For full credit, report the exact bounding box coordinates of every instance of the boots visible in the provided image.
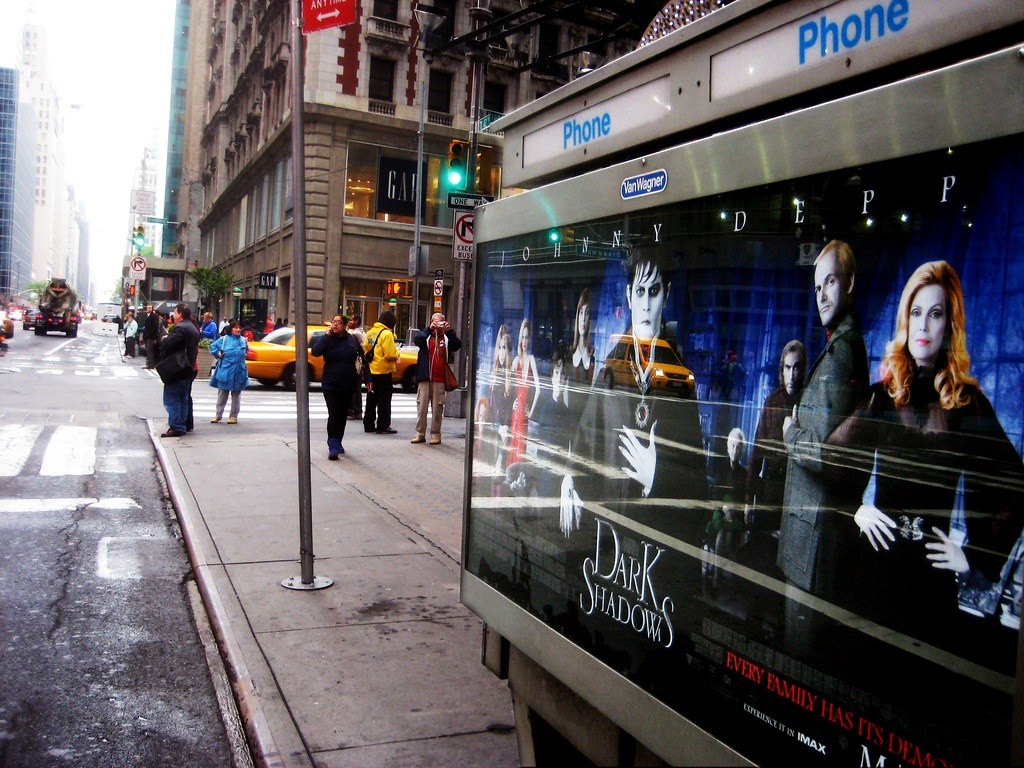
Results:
[327,438,338,459]
[337,441,344,454]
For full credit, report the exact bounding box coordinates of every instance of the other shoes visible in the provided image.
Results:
[227,418,237,423]
[365,427,377,432]
[346,414,362,420]
[430,438,441,444]
[410,437,426,443]
[211,416,221,423]
[376,424,397,434]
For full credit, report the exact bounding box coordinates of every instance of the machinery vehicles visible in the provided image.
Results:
[34,277,82,338]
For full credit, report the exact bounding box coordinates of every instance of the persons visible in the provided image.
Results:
[708,428,749,598]
[475,288,595,520]
[822,261,1024,613]
[219,317,234,335]
[199,312,217,341]
[263,317,288,333]
[363,311,400,434]
[311,314,372,460]
[209,320,249,423]
[411,313,462,445]
[142,305,159,369]
[347,313,366,420]
[775,241,871,656]
[561,235,707,613]
[123,309,138,358]
[160,303,202,438]
[746,340,807,578]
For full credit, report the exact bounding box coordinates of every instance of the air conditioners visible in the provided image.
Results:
[413,2,448,43]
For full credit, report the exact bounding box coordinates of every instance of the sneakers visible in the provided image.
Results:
[161,428,186,437]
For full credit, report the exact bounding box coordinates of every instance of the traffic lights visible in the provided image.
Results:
[131,226,138,245]
[549,231,560,244]
[391,280,407,295]
[446,142,464,187]
[561,227,575,245]
[137,224,145,247]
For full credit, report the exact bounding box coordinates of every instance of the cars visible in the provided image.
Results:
[0,310,15,343]
[22,308,38,330]
[245,323,421,393]
[102,314,121,324]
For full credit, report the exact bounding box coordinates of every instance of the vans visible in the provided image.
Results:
[599,333,696,399]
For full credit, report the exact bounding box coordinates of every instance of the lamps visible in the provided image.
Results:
[505,22,532,50]
[581,48,602,71]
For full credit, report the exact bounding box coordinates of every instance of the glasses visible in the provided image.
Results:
[330,320,344,324]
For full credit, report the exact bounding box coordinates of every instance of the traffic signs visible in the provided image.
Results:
[447,192,495,212]
[301,0,357,36]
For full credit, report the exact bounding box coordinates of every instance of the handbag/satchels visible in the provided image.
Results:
[444,363,457,391]
[156,351,192,384]
[209,365,219,388]
[350,333,362,375]
[365,349,374,362]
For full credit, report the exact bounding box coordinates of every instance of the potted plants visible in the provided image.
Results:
[194,339,214,379]
